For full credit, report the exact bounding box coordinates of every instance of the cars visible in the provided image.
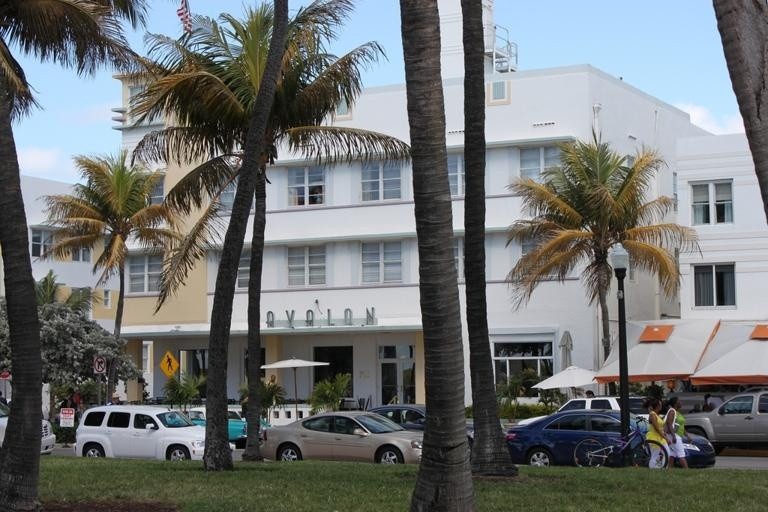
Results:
[167,405,268,443]
[1,395,56,455]
[259,411,424,464]
[370,405,473,445]
[507,398,717,468]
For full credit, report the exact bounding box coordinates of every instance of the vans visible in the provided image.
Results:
[74,404,236,462]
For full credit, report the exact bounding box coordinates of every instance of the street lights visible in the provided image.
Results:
[610,242,635,465]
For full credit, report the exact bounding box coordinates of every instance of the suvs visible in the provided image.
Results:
[684,387,767,457]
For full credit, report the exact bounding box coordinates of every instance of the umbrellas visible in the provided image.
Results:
[259,354,331,422]
[529,365,598,398]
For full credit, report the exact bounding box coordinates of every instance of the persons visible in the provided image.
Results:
[106,392,122,406]
[640,397,672,470]
[687,403,700,413]
[265,374,276,405]
[703,393,723,410]
[0,391,6,405]
[585,390,596,398]
[661,396,688,469]
